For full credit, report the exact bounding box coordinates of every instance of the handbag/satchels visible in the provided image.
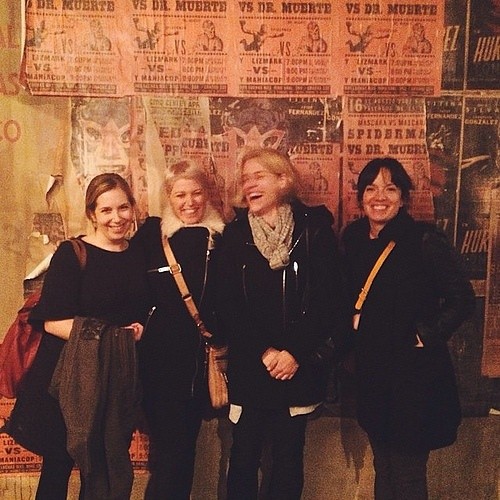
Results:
[285,335,340,419]
[334,330,370,410]
[0,236,89,400]
[208,346,229,409]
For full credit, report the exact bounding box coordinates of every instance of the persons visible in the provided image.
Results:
[51,162,227,500]
[328,157,476,500]
[217,148,338,500]
[4,173,146,500]
